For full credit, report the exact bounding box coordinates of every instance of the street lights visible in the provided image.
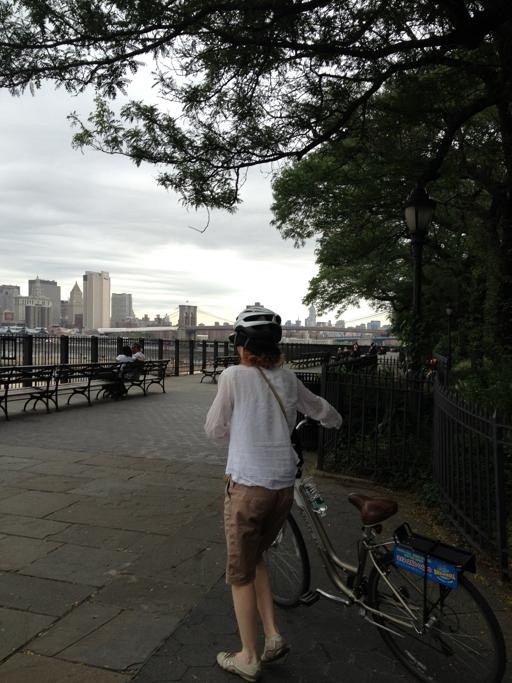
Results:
[444,301,455,390]
[402,178,436,433]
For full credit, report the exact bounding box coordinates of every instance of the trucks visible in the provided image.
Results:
[196,334,209,342]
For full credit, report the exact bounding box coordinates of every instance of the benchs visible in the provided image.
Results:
[0,358,171,421]
[289,347,388,369]
[201,354,239,383]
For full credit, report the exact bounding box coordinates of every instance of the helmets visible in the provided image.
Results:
[229,304,282,342]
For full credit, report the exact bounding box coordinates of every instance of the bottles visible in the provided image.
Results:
[299,475,329,519]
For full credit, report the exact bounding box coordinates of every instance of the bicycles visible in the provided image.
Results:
[260,415,506,682]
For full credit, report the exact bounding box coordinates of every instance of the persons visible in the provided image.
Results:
[336,340,388,353]
[202,302,346,681]
[101,344,133,399]
[131,342,145,363]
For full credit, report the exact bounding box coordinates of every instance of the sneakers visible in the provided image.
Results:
[216,651,262,682]
[260,634,290,664]
